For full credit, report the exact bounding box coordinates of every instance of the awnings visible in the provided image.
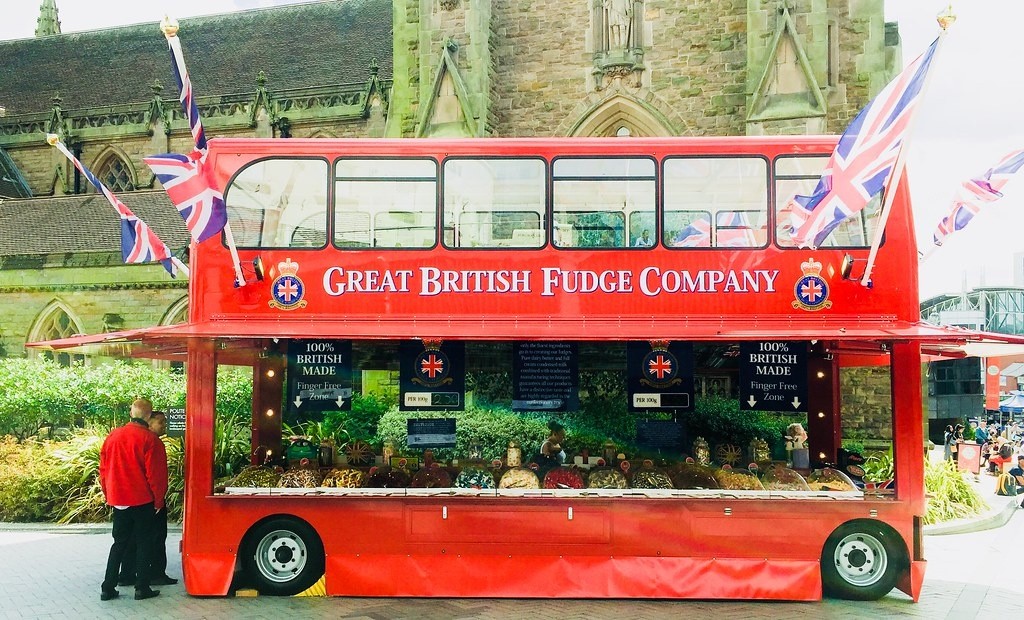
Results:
[144,319,1024,346]
[28,322,188,362]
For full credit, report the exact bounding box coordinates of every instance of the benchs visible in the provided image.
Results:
[1002,462,1017,476]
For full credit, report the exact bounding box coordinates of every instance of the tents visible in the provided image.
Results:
[985,395,1024,425]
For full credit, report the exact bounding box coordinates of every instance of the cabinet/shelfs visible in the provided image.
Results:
[956,443,982,474]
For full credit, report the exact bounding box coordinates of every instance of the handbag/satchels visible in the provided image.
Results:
[950,445,957,453]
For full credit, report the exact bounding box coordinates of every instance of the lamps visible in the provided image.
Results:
[841,253,867,282]
[240,255,265,281]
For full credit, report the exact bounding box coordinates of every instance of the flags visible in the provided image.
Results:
[778,188,804,246]
[142,74,228,244]
[674,211,753,247]
[789,36,939,250]
[935,149,1024,238]
[71,156,177,280]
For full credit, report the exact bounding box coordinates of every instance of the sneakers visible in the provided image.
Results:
[148,574,178,585]
[118,577,136,586]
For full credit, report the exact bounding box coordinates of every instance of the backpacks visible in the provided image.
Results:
[996,473,1017,495]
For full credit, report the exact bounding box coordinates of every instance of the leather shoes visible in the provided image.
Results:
[100,589,120,600]
[134,589,160,600]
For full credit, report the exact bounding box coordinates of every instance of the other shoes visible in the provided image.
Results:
[981,464,986,467]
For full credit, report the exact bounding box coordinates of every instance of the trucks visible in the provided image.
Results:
[24,134,927,603]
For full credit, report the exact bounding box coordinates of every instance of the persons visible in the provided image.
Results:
[980,420,1024,475]
[98,399,169,601]
[1008,455,1024,509]
[952,424,965,460]
[944,425,953,460]
[539,420,567,462]
[635,230,652,247]
[120,411,178,585]
[975,419,989,443]
